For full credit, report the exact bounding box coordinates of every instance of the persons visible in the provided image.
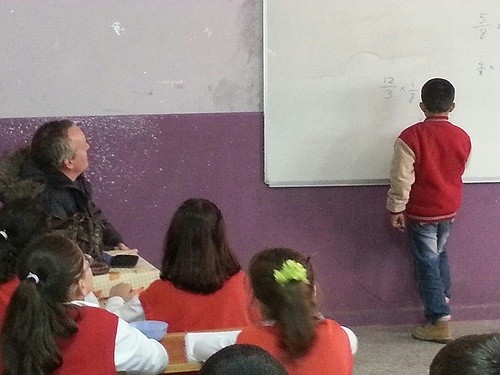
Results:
[0,233,168,375]
[192,247,358,375]
[106,198,267,335]
[198,344,290,375]
[386,77,472,343]
[1,119,139,277]
[0,196,101,327]
[429,332,500,375]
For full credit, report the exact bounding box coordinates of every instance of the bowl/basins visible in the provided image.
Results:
[129,320,169,342]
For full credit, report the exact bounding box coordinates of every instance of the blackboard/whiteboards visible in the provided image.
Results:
[263,0,500,184]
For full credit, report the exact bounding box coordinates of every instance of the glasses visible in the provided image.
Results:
[76,252,94,284]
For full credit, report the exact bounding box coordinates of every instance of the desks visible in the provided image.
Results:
[147,330,240,375]
[87,251,161,309]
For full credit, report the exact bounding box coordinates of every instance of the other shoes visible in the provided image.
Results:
[413,320,453,344]
